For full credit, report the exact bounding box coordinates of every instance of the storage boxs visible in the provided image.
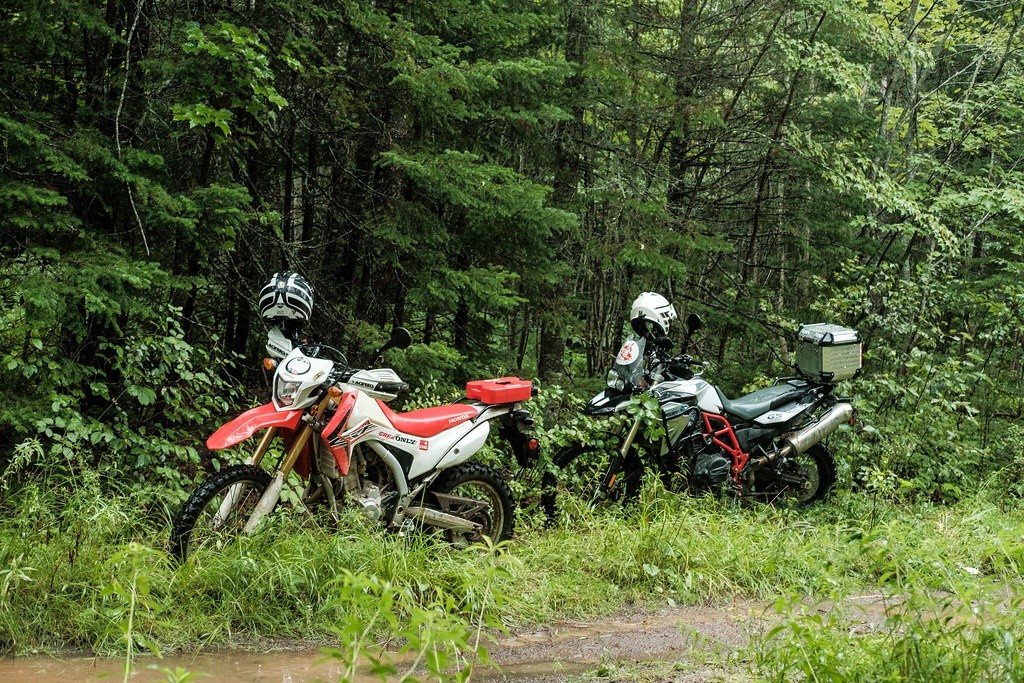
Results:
[795,322,862,381]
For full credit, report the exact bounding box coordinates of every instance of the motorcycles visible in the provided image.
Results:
[168,319,543,566]
[540,320,864,524]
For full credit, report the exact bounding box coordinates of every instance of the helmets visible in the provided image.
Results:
[629,291,678,343]
[258,270,315,330]
